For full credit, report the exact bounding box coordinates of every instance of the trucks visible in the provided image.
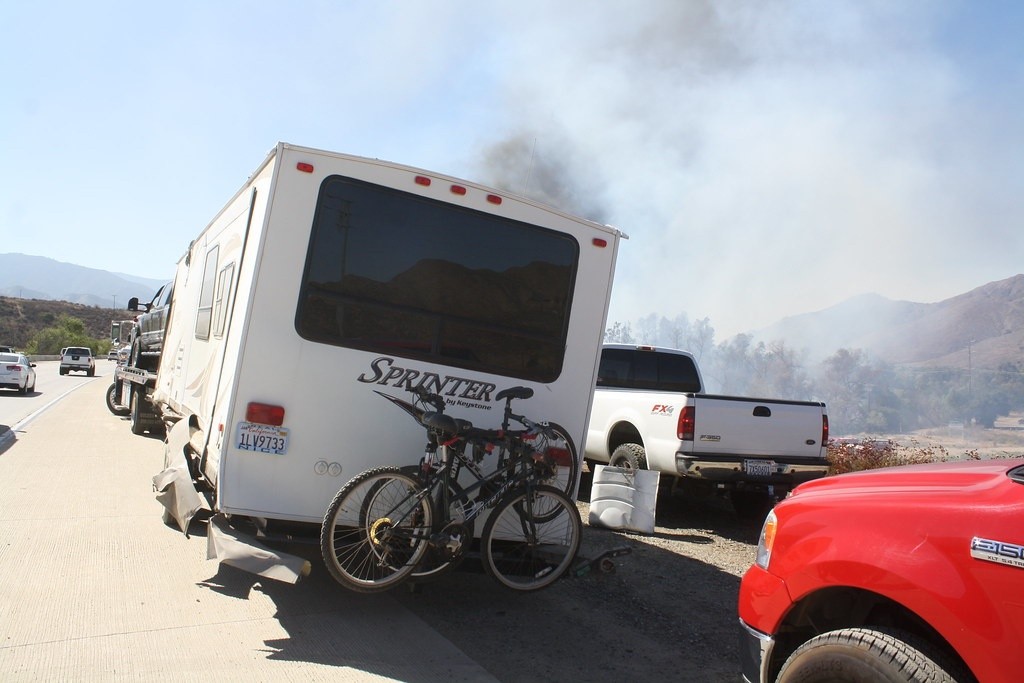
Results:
[105,314,166,435]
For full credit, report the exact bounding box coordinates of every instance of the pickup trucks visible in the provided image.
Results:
[59,346,96,377]
[581,341,832,513]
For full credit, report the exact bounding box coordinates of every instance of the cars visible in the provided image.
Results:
[127,281,176,368]
[0,351,37,394]
[108,348,118,361]
[735,450,1024,683]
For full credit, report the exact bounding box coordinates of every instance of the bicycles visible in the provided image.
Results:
[313,381,584,596]
